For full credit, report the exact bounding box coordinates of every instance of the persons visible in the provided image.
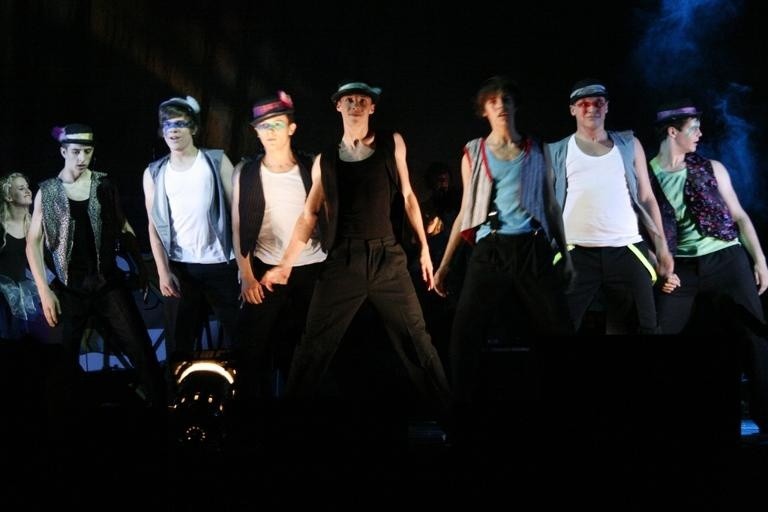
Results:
[0,172,42,344]
[231,90,445,414]
[257,77,453,412]
[543,81,681,345]
[137,94,241,382]
[434,76,568,405]
[24,117,159,377]
[644,97,767,413]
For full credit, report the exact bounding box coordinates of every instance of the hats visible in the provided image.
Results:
[569,83,608,104]
[61,125,97,147]
[332,80,382,104]
[656,106,701,124]
[251,98,292,126]
